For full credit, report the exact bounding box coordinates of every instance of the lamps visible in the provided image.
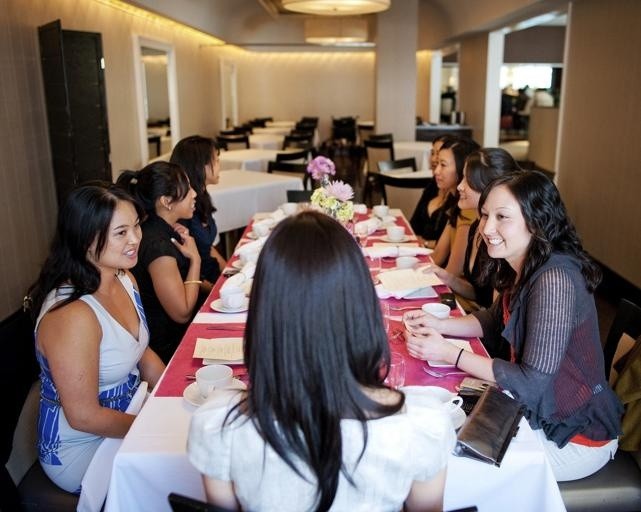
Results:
[280,0,391,15]
[305,16,369,44]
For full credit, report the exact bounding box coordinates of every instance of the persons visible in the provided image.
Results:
[185,211,457,511]
[402,169,623,482]
[116,160,215,364]
[410,134,451,224]
[169,133,228,279]
[26,183,167,494]
[417,137,480,268]
[417,148,522,309]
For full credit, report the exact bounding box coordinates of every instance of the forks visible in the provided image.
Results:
[423,366,468,378]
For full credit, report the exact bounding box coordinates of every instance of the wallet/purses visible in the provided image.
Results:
[452,384,528,468]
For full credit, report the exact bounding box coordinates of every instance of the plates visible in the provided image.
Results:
[378,235,409,241]
[402,286,438,298]
[202,338,244,365]
[210,297,250,312]
[183,378,247,407]
[232,260,259,269]
[246,232,272,239]
[427,339,474,367]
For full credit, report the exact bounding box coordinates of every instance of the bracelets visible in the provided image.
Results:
[425,239,428,247]
[455,348,464,368]
[184,281,203,284]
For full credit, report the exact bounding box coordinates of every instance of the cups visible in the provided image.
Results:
[279,202,297,216]
[396,256,417,269]
[365,256,382,285]
[252,223,268,236]
[384,353,408,414]
[358,223,369,247]
[219,286,244,308]
[378,300,389,333]
[239,249,258,267]
[422,303,450,320]
[387,227,404,240]
[374,205,388,217]
[195,365,233,400]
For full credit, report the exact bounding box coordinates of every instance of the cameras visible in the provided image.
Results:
[459,377,500,392]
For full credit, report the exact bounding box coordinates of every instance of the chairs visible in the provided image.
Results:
[558,299,639,512]
[289,188,313,202]
[277,150,308,163]
[168,492,478,512]
[1,304,79,512]
[330,115,360,144]
[370,171,431,222]
[217,116,320,147]
[369,133,392,143]
[304,169,316,189]
[268,159,308,178]
[376,157,418,173]
[364,139,395,172]
